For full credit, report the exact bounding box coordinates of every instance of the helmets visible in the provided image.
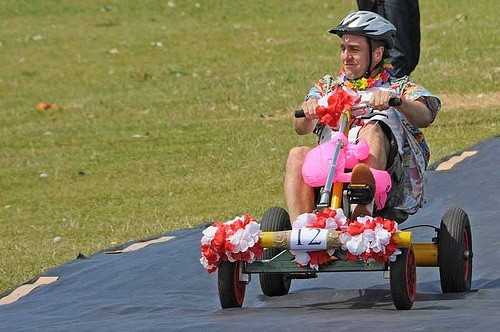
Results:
[328,10,397,50]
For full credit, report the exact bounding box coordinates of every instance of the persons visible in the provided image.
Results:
[280,11,442,267]
[355,0,421,81]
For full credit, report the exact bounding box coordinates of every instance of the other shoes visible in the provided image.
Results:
[349,162,376,225]
